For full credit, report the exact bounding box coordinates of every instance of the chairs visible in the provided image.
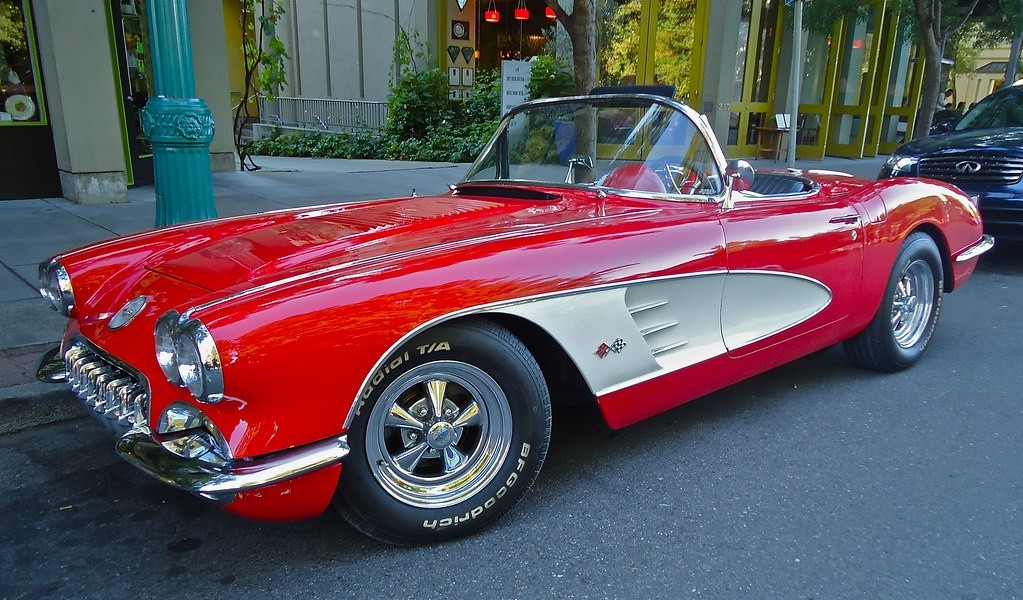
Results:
[602,163,669,194]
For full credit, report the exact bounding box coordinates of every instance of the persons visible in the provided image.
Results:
[935,89,976,110]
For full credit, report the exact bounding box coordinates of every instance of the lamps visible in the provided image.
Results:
[514,0,530,19]
[485,0,500,22]
[545,6,557,18]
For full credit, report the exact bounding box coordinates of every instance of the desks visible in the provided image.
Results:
[754,126,801,161]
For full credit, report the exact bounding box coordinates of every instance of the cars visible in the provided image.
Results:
[37,84,999,548]
[879,78,1023,240]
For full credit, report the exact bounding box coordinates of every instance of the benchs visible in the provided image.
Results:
[650,155,807,195]
